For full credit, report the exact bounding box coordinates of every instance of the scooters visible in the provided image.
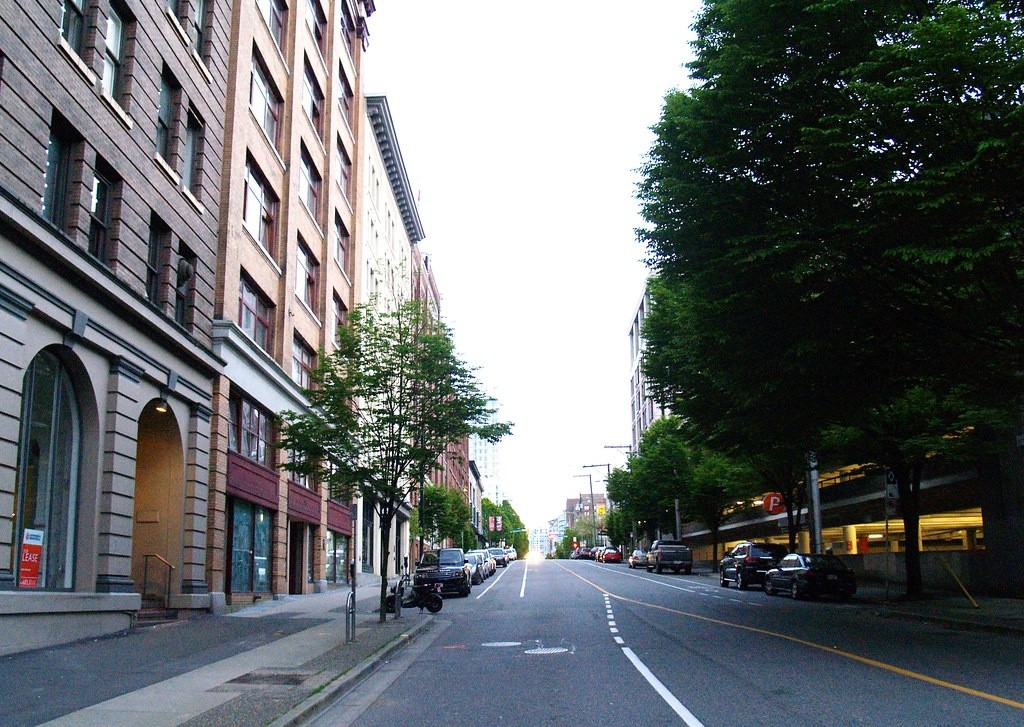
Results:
[385,574,443,615]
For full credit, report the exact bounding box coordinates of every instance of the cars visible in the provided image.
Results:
[571,546,623,564]
[628,549,649,568]
[763,552,858,601]
[464,545,518,585]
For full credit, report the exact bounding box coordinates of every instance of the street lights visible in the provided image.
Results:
[583,463,614,545]
[573,473,596,546]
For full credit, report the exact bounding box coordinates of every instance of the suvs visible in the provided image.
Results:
[718,540,790,592]
[646,539,694,575]
[412,547,471,598]
